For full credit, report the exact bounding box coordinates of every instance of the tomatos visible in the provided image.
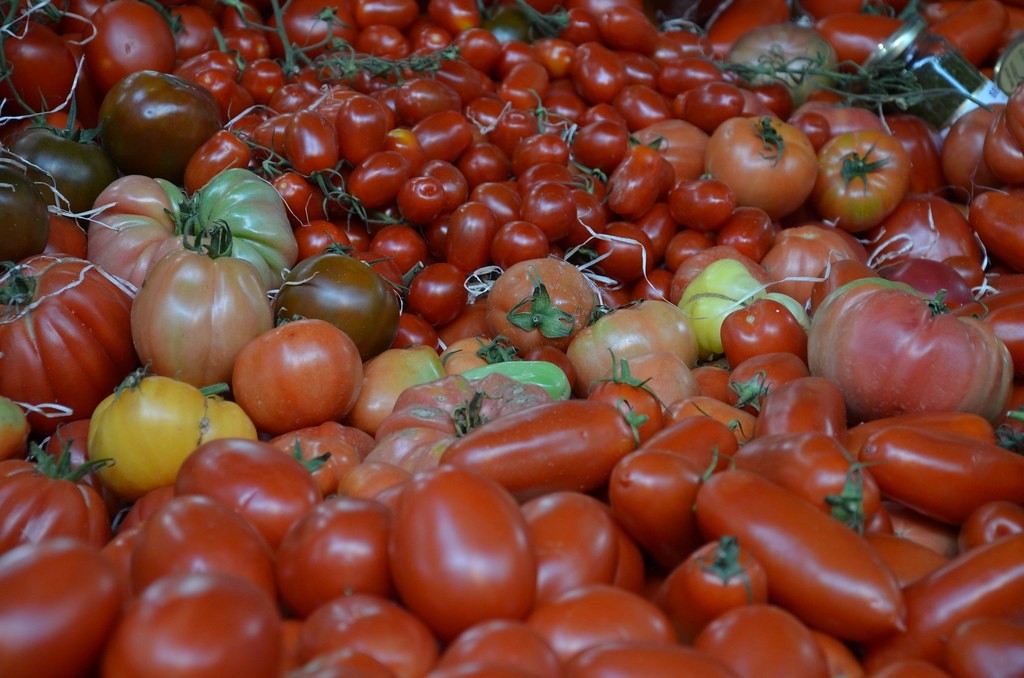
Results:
[0,0,1024,678]
